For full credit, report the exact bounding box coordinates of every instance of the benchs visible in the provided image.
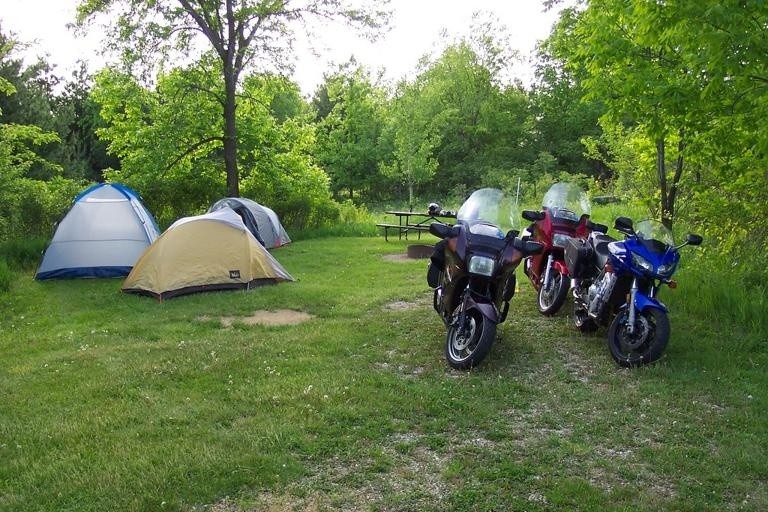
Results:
[375,223,429,242]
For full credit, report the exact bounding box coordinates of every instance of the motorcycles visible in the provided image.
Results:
[520,179,609,315]
[561,215,704,368]
[420,186,542,374]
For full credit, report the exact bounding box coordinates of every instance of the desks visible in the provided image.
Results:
[384,211,457,240]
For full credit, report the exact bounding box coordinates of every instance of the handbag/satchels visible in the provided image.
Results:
[564,235,594,280]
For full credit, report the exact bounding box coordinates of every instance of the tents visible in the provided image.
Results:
[202,195,292,251]
[119,207,298,303]
[30,181,162,283]
